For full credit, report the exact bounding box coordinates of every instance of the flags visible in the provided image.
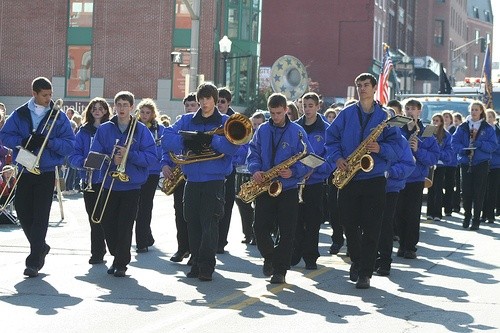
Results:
[378,52,394,104]
[483,44,493,111]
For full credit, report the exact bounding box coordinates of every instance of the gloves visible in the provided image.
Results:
[191,131,211,144]
[465,150,470,155]
[27,135,46,150]
[471,140,481,149]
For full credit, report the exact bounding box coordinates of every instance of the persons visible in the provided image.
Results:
[324,73,404,288]
[87,91,158,277]
[246,93,315,285]
[0,102,19,228]
[161,81,248,281]
[217,84,356,262]
[0,77,76,278]
[385,101,500,259]
[67,93,200,263]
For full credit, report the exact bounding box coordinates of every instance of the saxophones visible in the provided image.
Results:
[159,162,189,196]
[236,130,326,205]
[332,99,393,190]
[407,114,421,149]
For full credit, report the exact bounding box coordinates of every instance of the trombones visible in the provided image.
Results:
[0,98,63,210]
[90,107,142,224]
[55,165,65,220]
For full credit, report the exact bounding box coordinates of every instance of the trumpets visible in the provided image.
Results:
[85,131,96,193]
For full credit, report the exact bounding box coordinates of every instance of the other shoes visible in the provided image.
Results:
[188,256,192,265]
[187,267,199,276]
[199,270,211,280]
[23,266,38,277]
[136,246,147,253]
[170,248,190,262]
[426,203,499,230]
[107,264,116,274]
[69,189,76,195]
[38,244,50,271]
[89,256,103,263]
[216,247,223,254]
[113,266,125,276]
[242,236,417,289]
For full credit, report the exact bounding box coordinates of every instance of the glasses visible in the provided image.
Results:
[218,100,227,103]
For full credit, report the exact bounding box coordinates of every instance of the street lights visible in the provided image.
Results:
[218,34,233,92]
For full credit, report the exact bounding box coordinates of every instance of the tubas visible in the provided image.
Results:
[166,112,254,165]
